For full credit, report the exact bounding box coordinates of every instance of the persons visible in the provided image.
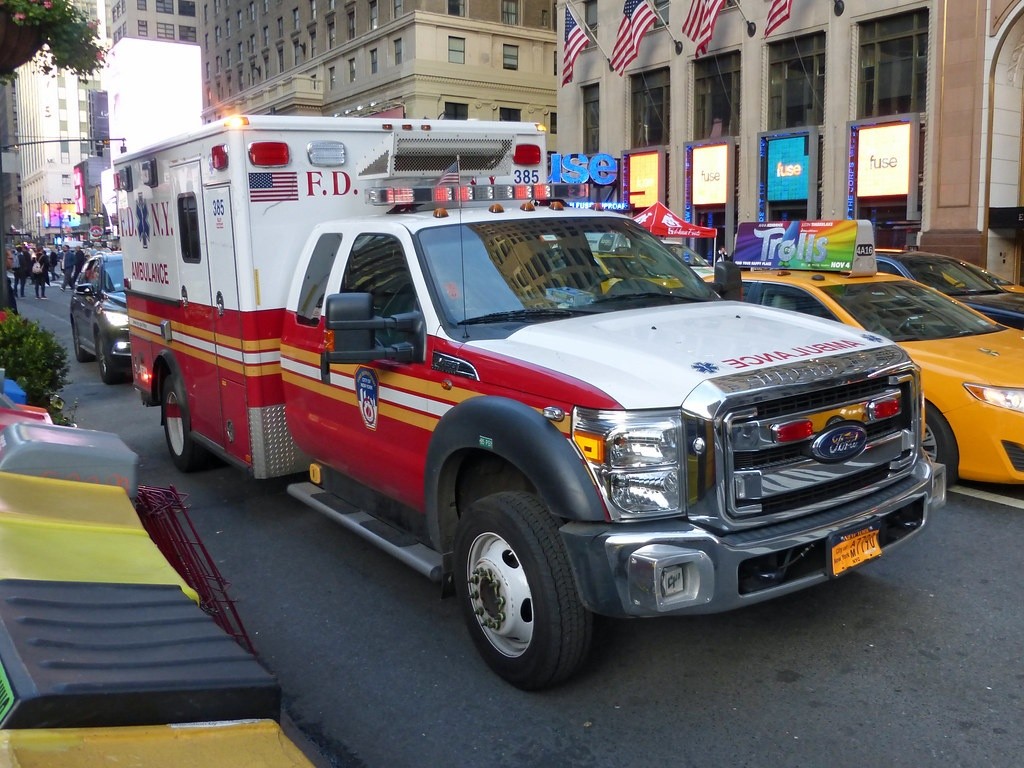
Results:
[5,247,19,315]
[14,246,60,300]
[60,245,85,292]
[716,247,728,263]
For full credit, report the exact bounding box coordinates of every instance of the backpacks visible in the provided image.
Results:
[32,255,44,274]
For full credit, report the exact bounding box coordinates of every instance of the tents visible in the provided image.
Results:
[610,202,717,283]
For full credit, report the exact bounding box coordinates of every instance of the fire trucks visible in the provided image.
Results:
[112,116,947,687]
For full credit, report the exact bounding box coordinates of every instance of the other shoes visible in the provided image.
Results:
[21,296,27,299]
[14,295,18,300]
[60,288,65,293]
[35,296,48,300]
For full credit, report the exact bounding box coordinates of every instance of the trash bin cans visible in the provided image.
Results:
[1,366,316,767]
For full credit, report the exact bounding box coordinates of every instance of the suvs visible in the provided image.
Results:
[69,252,133,383]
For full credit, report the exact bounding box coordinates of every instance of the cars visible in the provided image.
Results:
[699,220,1024,487]
[875,252,1024,329]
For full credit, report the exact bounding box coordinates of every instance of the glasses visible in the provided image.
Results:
[8,257,14,260]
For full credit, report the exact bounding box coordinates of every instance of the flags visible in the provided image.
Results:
[764,0,792,41]
[562,4,590,88]
[610,0,657,78]
[682,0,727,58]
[436,160,459,186]
